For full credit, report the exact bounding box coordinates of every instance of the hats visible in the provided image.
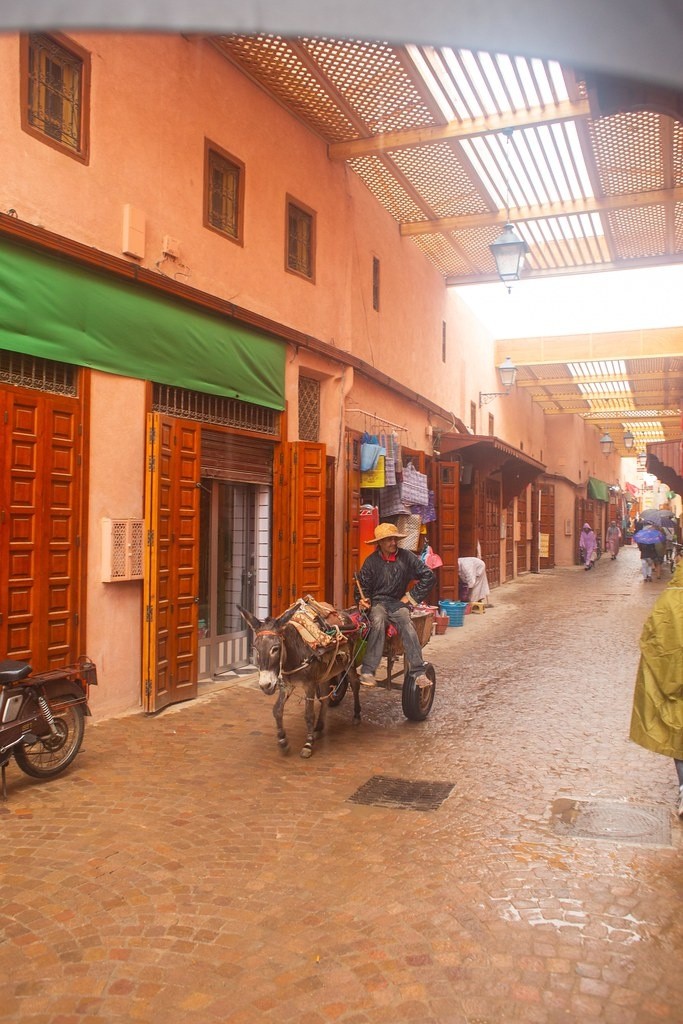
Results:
[365,523,407,544]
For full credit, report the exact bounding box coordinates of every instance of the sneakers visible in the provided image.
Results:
[416,675,433,688]
[359,674,377,686]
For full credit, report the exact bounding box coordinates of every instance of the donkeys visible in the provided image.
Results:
[236,602,363,758]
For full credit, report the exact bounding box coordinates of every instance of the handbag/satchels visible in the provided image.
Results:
[380,462,436,552]
[360,430,403,487]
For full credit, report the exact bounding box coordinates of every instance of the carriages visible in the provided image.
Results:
[235,595,436,758]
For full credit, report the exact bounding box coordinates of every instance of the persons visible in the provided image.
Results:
[606,521,622,560]
[629,561,683,815]
[628,517,675,581]
[579,523,597,571]
[353,523,436,689]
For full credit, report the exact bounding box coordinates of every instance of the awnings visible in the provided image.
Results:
[432,430,547,509]
[644,439,683,498]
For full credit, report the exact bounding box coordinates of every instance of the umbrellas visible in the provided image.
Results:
[640,509,679,528]
[632,529,668,544]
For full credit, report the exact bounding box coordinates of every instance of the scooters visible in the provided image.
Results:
[0,655,98,803]
